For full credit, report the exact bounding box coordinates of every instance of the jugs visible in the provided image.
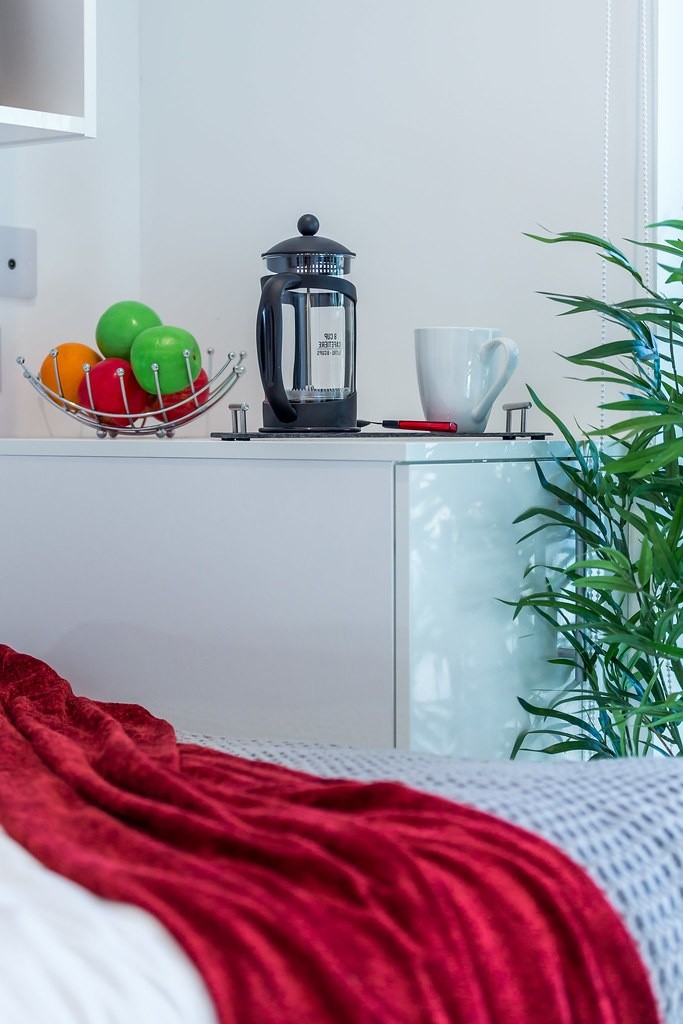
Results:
[256,213,361,434]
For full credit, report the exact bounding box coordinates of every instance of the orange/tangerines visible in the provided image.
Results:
[40,343,103,411]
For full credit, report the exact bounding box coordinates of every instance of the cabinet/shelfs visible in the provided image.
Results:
[0,437,658,763]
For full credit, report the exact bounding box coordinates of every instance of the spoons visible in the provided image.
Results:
[356,419,458,433]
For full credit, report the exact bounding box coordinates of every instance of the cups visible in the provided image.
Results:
[415,328,520,434]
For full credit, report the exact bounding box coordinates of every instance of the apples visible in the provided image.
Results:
[76,300,210,425]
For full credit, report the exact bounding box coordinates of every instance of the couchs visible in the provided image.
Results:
[0,685,683,1024]
